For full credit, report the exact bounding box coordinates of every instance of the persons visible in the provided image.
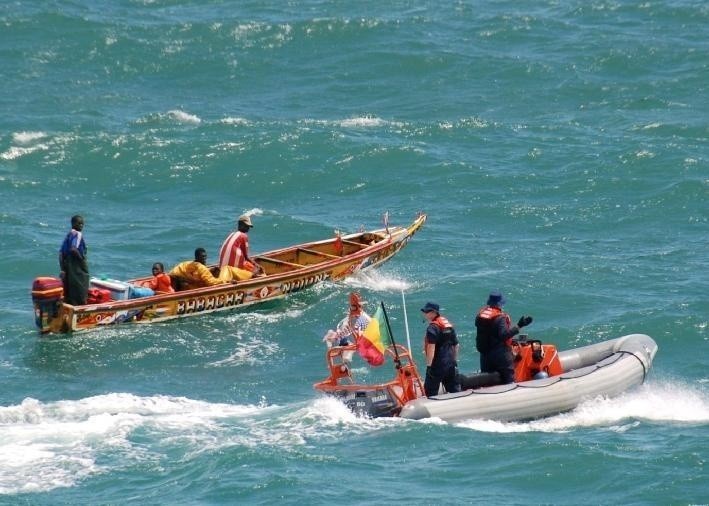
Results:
[148,262,175,293]
[217,214,266,281]
[57,214,91,307]
[418,300,459,396]
[167,247,261,290]
[474,290,534,385]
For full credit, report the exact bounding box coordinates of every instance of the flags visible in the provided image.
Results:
[357,306,391,366]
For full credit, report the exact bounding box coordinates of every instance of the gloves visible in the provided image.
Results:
[518,315,532,328]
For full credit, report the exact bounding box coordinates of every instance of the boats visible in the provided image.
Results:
[328,330,661,431]
[29,208,430,336]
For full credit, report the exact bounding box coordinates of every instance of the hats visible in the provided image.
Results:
[420,301,439,313]
[239,215,253,227]
[486,291,507,305]
